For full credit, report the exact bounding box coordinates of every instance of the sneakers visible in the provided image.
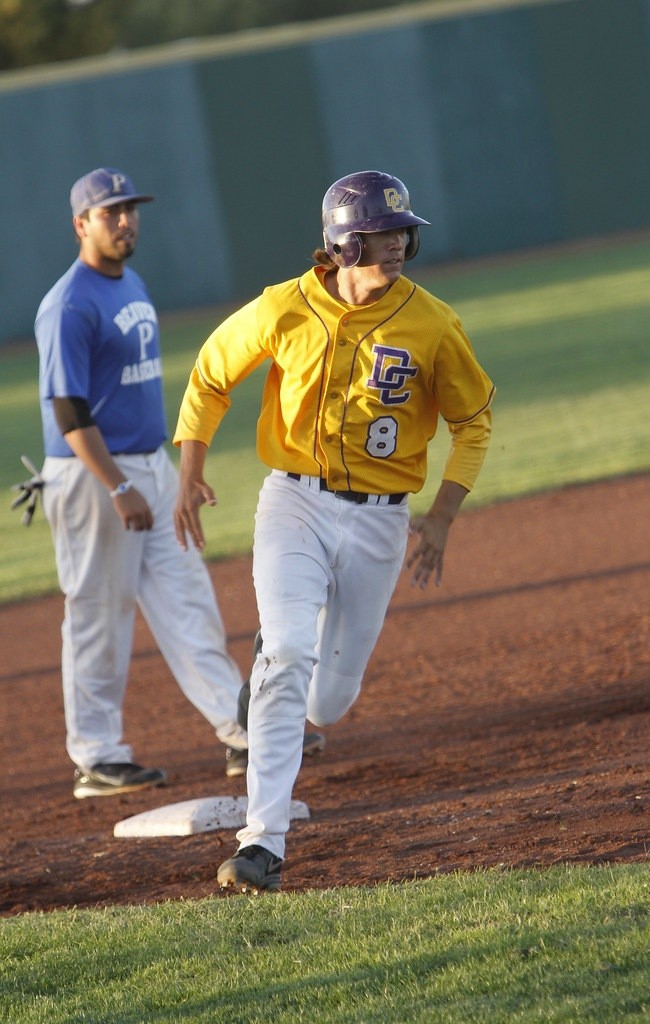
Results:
[216,845,283,895]
[73,764,165,798]
[235,627,264,730]
[228,731,325,776]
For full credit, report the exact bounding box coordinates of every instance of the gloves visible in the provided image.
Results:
[10,455,45,526]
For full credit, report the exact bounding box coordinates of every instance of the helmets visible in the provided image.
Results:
[320,171,431,268]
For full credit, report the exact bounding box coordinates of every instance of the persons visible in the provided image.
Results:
[171,171,496,893]
[38,169,325,798]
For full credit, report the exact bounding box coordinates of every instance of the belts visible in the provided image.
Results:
[287,472,406,504]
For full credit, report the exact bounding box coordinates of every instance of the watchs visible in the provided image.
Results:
[110,479,134,499]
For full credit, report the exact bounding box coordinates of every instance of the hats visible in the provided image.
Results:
[68,167,154,215]
